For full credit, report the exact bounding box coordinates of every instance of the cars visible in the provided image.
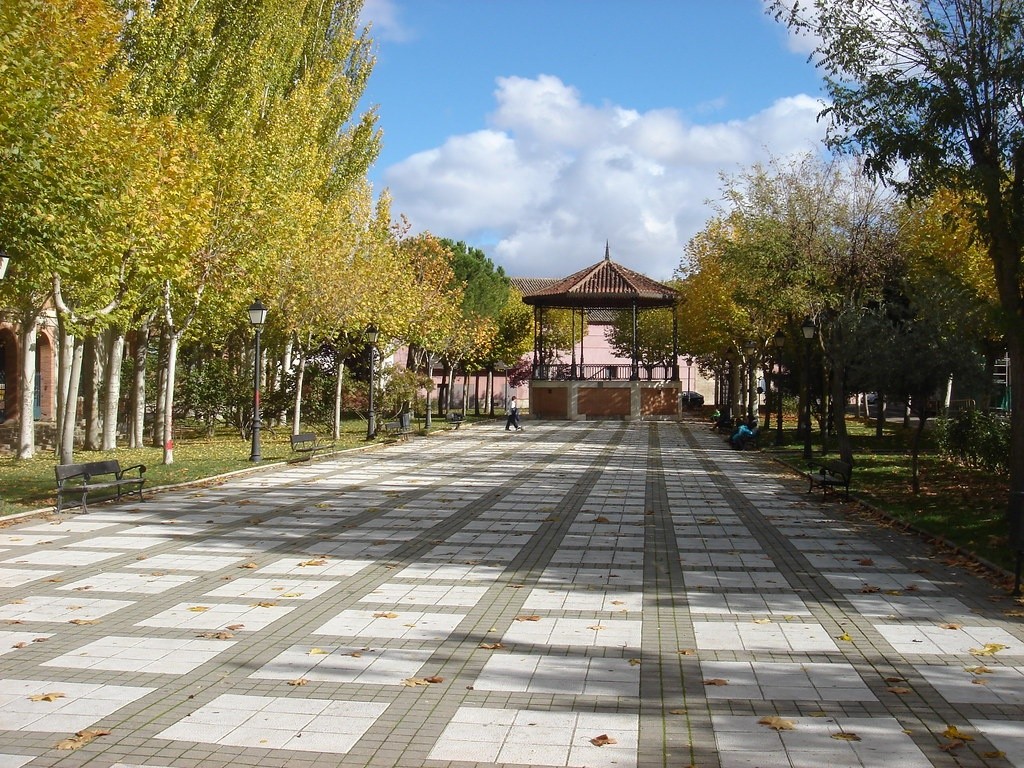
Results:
[682,391,704,408]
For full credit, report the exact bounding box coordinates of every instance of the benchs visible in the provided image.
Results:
[806,460,852,503]
[287,433,335,465]
[719,419,735,434]
[385,423,415,442]
[744,430,762,451]
[1005,491,1024,595]
[56,459,146,515]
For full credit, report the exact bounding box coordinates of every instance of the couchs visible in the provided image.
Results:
[446,413,468,431]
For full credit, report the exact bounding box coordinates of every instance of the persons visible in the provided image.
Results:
[725,418,759,451]
[709,406,730,434]
[505,396,522,431]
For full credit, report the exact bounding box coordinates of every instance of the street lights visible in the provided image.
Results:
[773,327,786,446]
[800,316,817,460]
[247,297,268,464]
[744,338,756,431]
[686,357,693,411]
[727,347,734,434]
[365,324,379,441]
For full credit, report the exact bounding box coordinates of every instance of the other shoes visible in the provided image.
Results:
[709,428,715,431]
[506,428,511,431]
